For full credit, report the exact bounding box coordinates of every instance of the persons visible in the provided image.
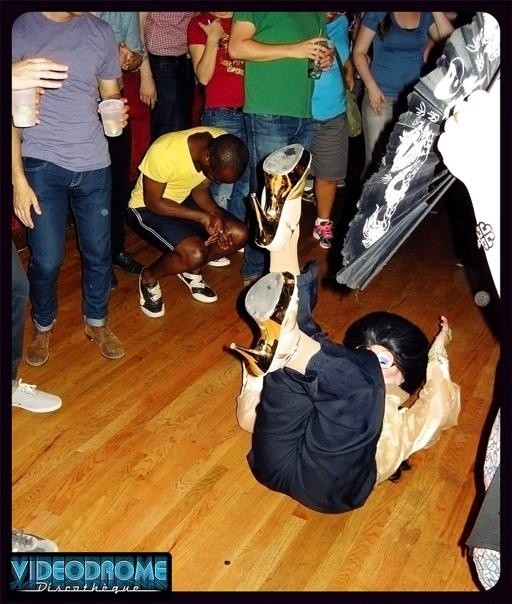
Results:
[436,81,501,592]
[11,11,130,367]
[227,144,461,516]
[97,11,493,319]
[11,57,68,413]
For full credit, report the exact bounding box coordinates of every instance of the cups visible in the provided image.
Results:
[307,58,322,79]
[97,99,124,138]
[12,89,36,128]
[327,39,335,64]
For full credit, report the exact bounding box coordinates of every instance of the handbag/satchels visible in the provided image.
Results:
[117,42,144,72]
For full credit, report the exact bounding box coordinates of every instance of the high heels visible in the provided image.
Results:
[229,270,300,377]
[251,143,312,250]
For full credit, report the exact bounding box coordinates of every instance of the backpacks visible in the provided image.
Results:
[344,89,365,138]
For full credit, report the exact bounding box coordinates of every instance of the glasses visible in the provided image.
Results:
[354,345,397,369]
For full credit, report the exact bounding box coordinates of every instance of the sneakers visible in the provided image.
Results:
[207,256,231,267]
[176,271,218,303]
[139,265,165,318]
[313,217,334,250]
[12,378,63,413]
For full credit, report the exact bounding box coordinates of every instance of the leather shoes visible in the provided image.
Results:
[112,248,146,276]
[84,323,126,360]
[25,324,53,367]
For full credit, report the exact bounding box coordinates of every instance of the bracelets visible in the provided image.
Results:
[427,345,448,358]
